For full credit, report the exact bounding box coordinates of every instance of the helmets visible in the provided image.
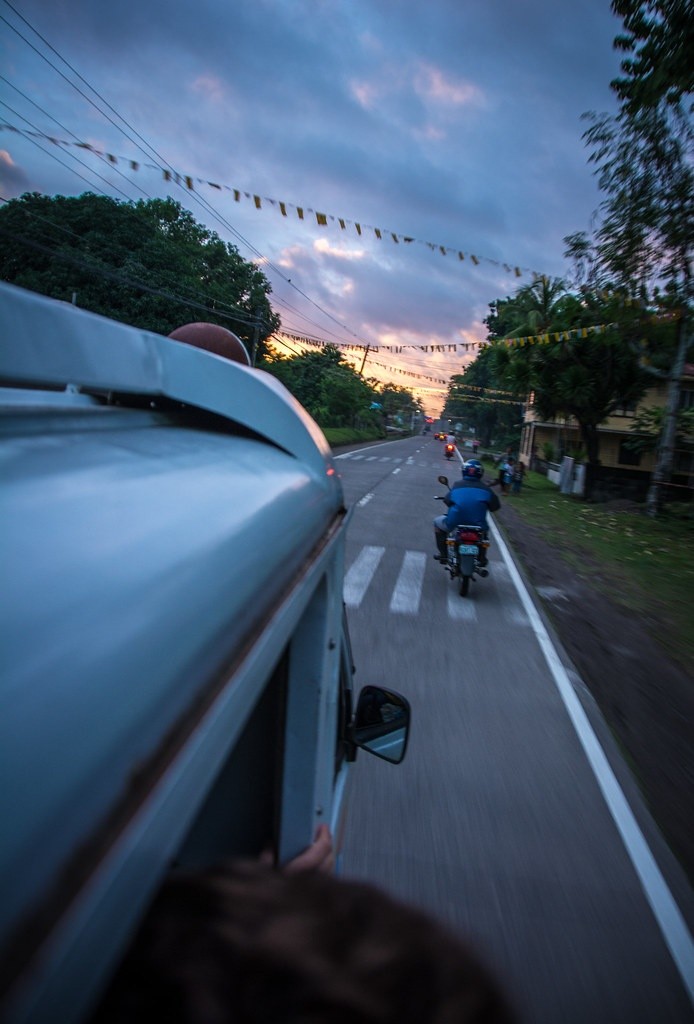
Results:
[449,430,455,435]
[462,458,484,481]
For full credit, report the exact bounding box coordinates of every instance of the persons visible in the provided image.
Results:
[89,822,521,1024]
[432,459,501,568]
[472,439,480,453]
[492,447,526,497]
[357,687,405,727]
[443,431,457,458]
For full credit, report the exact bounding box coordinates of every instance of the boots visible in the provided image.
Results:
[478,538,488,565]
[433,531,448,564]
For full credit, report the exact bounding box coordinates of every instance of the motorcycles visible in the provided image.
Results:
[434,476,499,597]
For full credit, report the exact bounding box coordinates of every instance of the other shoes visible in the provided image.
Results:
[512,492,519,496]
[501,492,508,496]
